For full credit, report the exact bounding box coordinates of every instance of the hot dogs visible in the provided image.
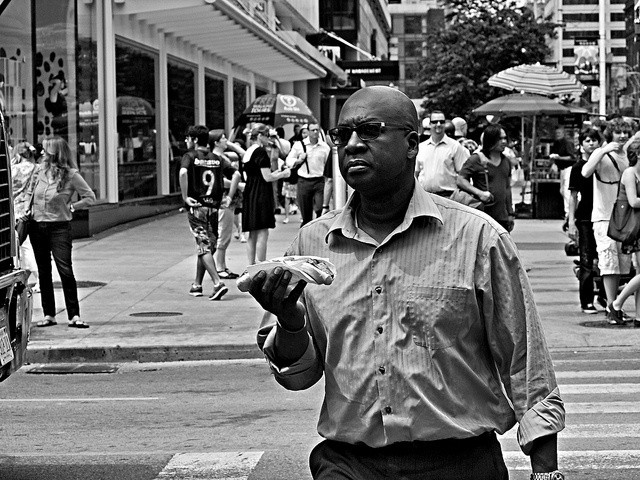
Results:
[236,256,336,292]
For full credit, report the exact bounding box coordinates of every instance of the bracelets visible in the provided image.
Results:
[276,313,308,333]
[322,204,329,209]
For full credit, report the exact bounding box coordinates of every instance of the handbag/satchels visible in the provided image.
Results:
[450,187,489,212]
[15,211,36,246]
[607,177,640,242]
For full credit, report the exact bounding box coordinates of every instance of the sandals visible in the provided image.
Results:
[217,268,239,279]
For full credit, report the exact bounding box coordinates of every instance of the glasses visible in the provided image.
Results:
[329,121,412,145]
[430,120,445,124]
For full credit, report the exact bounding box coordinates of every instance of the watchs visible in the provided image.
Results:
[70,204,75,212]
[530,470,565,479]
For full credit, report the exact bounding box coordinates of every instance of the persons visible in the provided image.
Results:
[295,127,309,141]
[206,129,246,279]
[179,124,241,302]
[456,124,514,234]
[10,141,41,293]
[443,119,457,143]
[418,118,431,143]
[320,141,348,216]
[609,138,640,327]
[285,122,332,228]
[290,125,301,144]
[281,147,306,224]
[566,128,607,314]
[270,126,297,214]
[47,75,74,148]
[452,116,479,155]
[18,137,96,328]
[502,147,523,205]
[242,126,291,265]
[581,118,634,324]
[549,127,577,232]
[250,84,566,479]
[413,110,472,206]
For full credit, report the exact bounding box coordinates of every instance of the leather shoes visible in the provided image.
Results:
[610,302,625,325]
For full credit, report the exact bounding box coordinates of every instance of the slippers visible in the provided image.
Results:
[68,320,89,328]
[37,319,57,327]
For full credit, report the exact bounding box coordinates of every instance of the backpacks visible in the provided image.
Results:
[281,164,298,184]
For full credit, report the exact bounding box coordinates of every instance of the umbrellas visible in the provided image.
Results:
[472,92,571,206]
[487,62,588,168]
[115,95,155,135]
[232,92,318,127]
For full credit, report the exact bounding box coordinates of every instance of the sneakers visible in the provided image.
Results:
[605,306,632,321]
[209,282,228,300]
[583,303,598,314]
[188,283,203,297]
